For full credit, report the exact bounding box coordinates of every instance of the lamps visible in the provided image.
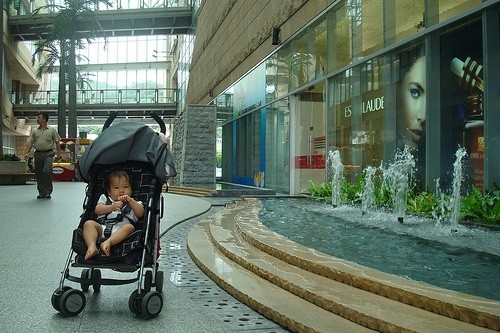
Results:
[271,27,280,45]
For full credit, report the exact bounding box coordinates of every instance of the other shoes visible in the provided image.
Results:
[37,193,51,199]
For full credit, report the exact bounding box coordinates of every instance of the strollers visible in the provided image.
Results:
[50,112,178,320]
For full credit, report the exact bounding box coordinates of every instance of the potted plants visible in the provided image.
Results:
[0,152,26,173]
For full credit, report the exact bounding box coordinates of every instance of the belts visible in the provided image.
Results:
[36,149,52,152]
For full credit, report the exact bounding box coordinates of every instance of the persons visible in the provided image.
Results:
[58,143,71,162]
[25,112,61,199]
[397,40,427,152]
[83,166,146,260]
